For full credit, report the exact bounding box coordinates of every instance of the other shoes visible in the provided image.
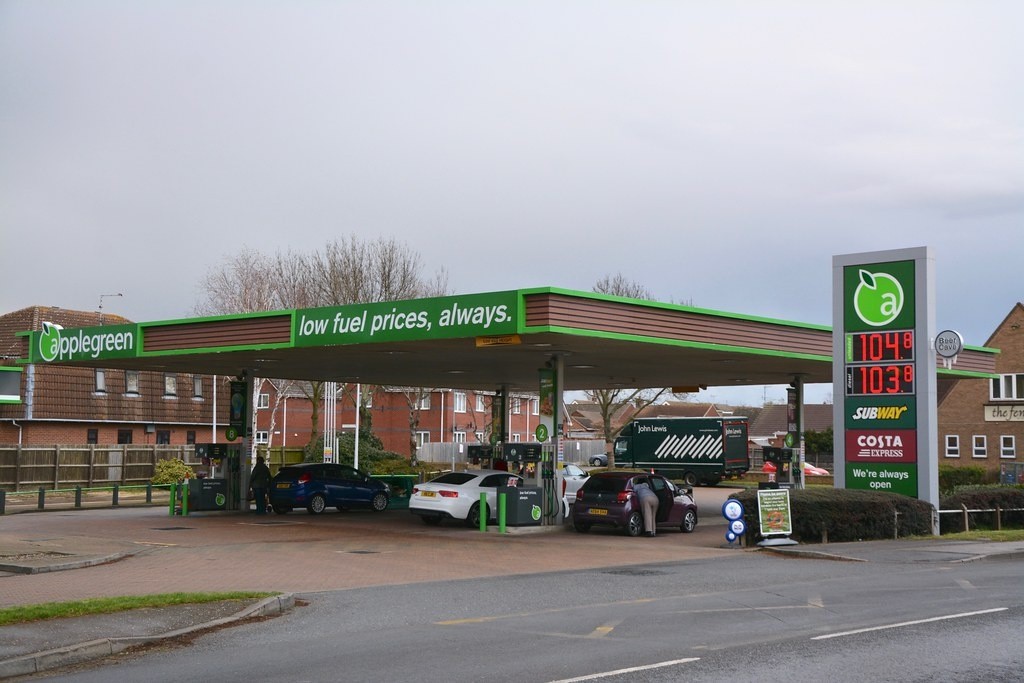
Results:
[646,533,654,537]
[256,511,266,515]
[642,531,651,536]
[250,509,258,513]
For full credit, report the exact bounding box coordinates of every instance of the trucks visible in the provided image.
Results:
[614,417,751,486]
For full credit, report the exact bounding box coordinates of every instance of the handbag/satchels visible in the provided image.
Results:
[247,485,257,501]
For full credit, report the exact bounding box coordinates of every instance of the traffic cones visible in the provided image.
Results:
[650,468,654,474]
[176,472,194,511]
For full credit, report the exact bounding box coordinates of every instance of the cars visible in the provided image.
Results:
[407,468,570,529]
[763,460,831,476]
[590,449,626,467]
[563,464,591,504]
[573,471,698,536]
[268,463,392,515]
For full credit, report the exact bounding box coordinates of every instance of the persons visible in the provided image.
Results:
[250,457,272,511]
[633,483,659,536]
